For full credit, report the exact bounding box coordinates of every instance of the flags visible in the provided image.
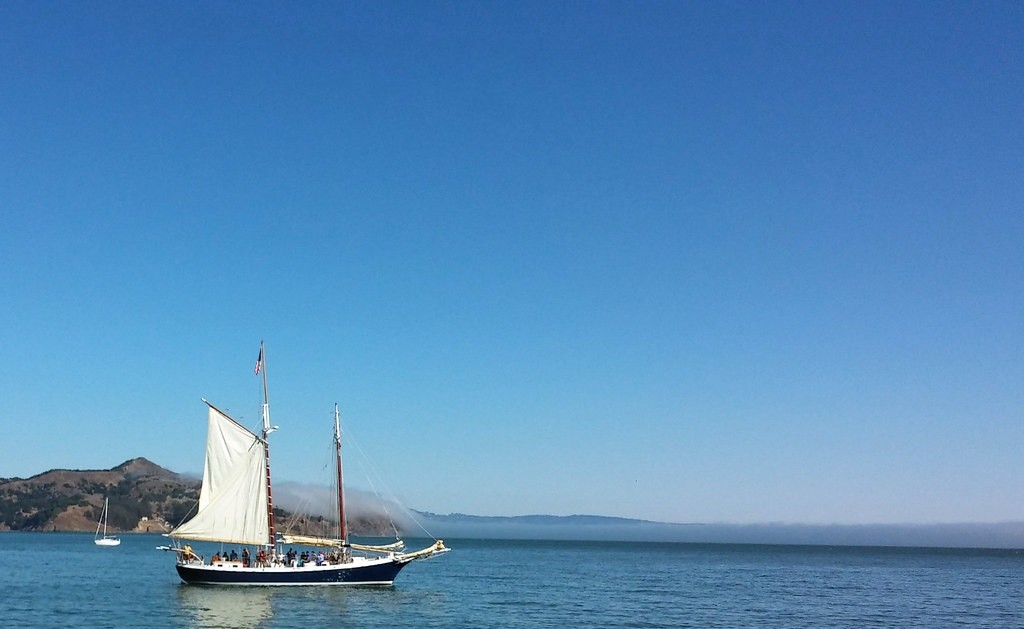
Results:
[254,349,262,375]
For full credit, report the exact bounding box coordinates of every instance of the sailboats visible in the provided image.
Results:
[155,340,451,588]
[94,497,120,545]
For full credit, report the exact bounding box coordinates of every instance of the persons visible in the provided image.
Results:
[214,547,325,568]
[184,543,192,560]
